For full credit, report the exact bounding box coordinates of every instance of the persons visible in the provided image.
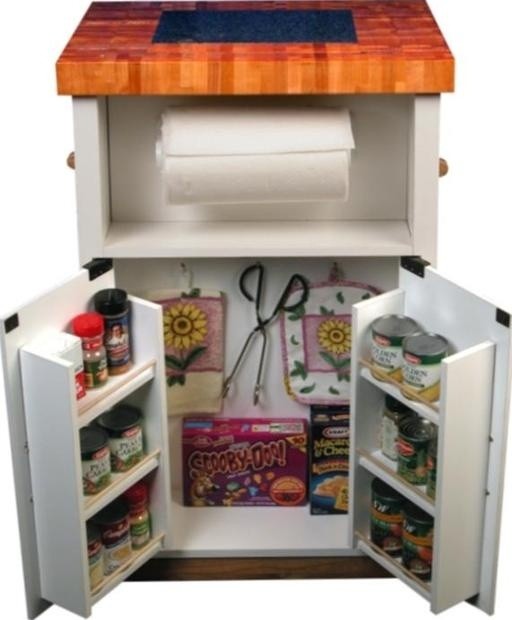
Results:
[106,323,130,345]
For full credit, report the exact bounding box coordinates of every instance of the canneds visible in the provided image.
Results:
[73,302,152,591]
[370,314,450,582]
